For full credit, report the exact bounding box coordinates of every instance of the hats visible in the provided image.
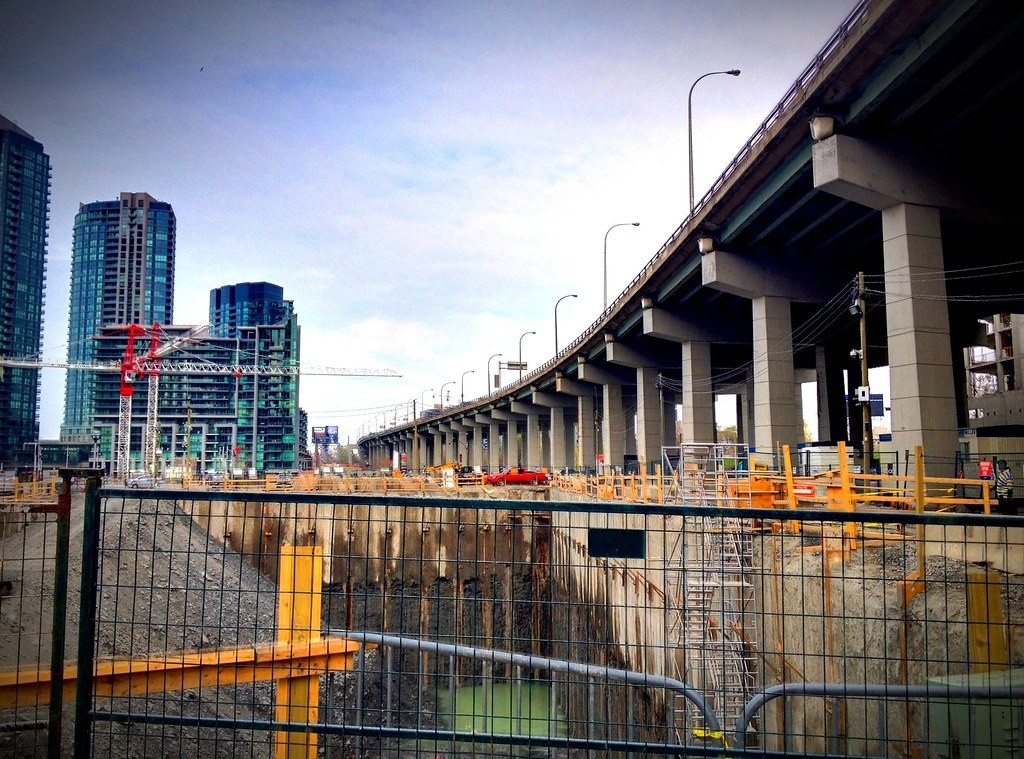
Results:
[995,460,1007,465]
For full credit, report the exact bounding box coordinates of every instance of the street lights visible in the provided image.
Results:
[440,381,455,411]
[602,221,640,310]
[90,429,103,468]
[358,398,413,438]
[554,294,578,355]
[685,68,741,217]
[422,388,434,411]
[487,352,502,398]
[461,370,474,402]
[518,331,536,383]
[849,299,876,505]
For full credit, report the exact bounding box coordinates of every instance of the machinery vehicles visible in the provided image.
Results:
[423,462,488,479]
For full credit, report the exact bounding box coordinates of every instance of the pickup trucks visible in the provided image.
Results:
[490,467,547,486]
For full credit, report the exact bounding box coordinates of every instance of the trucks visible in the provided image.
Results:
[200,462,392,481]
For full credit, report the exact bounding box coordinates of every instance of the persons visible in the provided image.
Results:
[995,458,1014,515]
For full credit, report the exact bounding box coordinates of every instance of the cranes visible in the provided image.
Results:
[0,323,401,483]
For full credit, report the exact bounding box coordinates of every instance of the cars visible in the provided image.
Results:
[125,473,159,489]
[545,467,566,477]
[547,468,580,480]
